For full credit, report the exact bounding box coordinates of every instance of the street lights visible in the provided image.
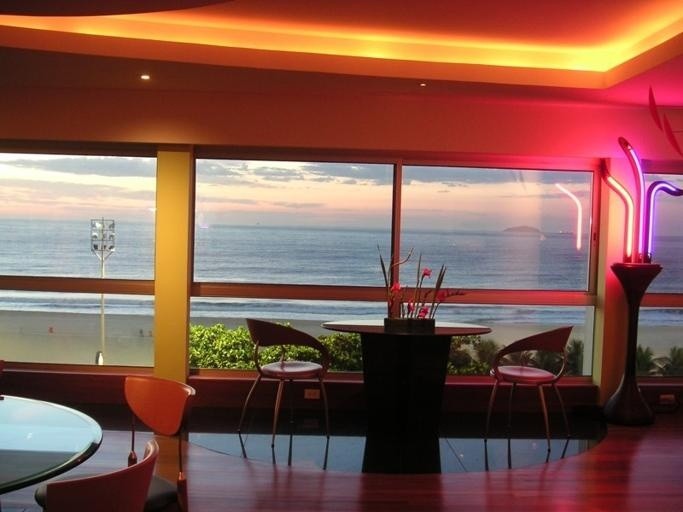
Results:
[91,217,116,352]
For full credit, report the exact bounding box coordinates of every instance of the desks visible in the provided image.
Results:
[0,395,104,496]
[320,320,492,485]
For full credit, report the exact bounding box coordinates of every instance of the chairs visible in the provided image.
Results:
[484,324,576,453]
[33,373,197,512]
[235,317,334,449]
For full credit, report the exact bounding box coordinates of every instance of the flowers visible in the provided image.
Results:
[372,245,467,321]
